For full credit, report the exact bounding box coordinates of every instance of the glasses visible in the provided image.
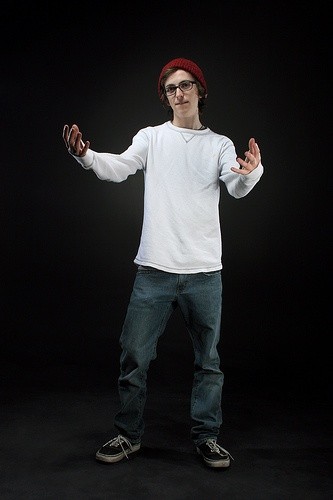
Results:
[163,81,196,96]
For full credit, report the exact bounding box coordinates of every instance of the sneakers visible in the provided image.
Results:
[195,440,231,466]
[95,436,141,462]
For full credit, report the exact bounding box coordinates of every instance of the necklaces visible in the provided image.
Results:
[175,124,204,144]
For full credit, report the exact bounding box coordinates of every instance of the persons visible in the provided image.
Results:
[62,59,265,469]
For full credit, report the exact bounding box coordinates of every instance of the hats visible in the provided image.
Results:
[158,58,207,93]
